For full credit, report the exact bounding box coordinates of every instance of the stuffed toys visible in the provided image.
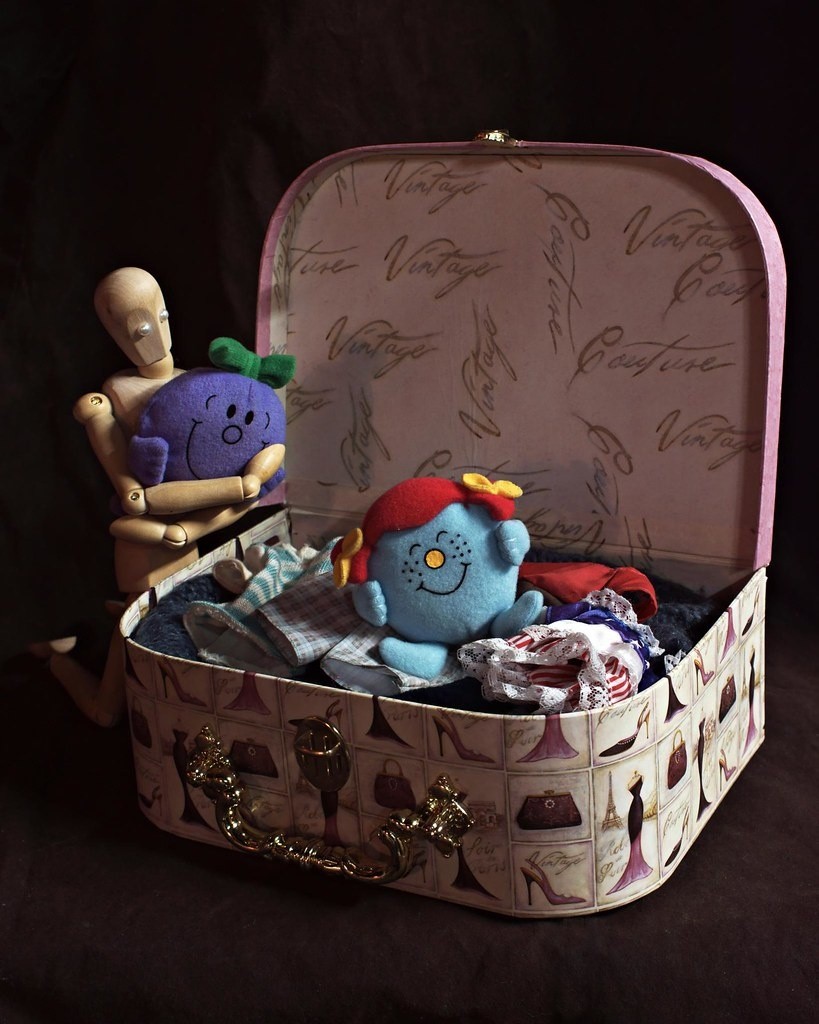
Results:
[322,471,552,681]
[123,338,299,512]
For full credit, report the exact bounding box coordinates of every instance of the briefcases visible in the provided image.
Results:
[120,131,787,919]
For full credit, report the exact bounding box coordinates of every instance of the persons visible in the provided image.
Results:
[24,264,289,730]
